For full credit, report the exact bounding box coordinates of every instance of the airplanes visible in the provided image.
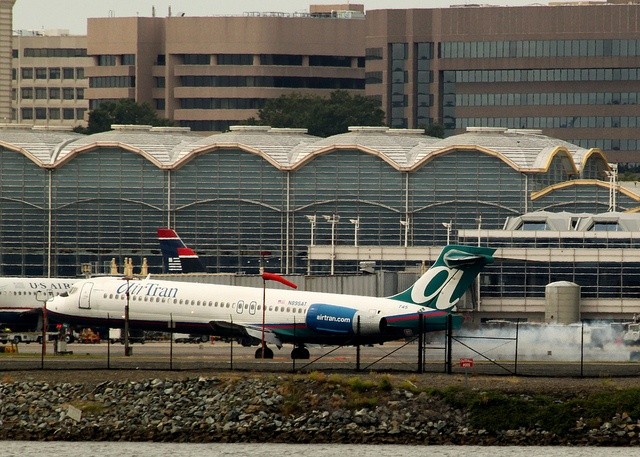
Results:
[1,229,208,320]
[46,245,497,358]
